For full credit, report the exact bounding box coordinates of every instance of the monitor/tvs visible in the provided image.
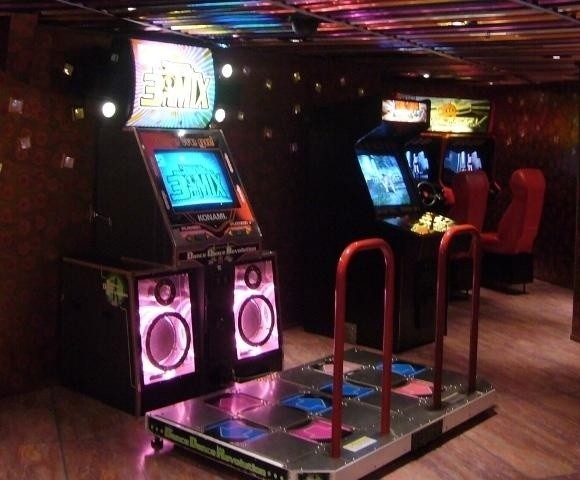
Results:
[407,147,430,180]
[444,152,483,174]
[149,146,242,214]
[356,151,412,211]
